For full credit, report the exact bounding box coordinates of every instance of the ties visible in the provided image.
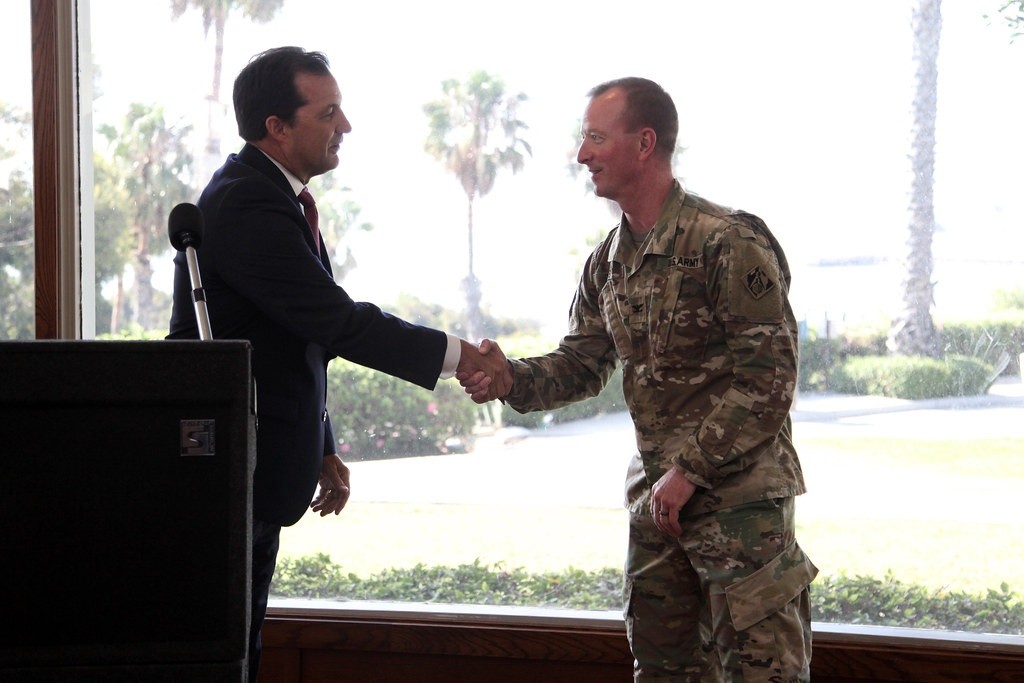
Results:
[298,186,320,257]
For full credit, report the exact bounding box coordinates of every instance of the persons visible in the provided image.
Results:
[455,76,820,683]
[167,48,512,683]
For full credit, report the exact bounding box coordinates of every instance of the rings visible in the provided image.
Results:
[657,510,668,517]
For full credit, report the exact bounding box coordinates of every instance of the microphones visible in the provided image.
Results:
[168,203,212,341]
